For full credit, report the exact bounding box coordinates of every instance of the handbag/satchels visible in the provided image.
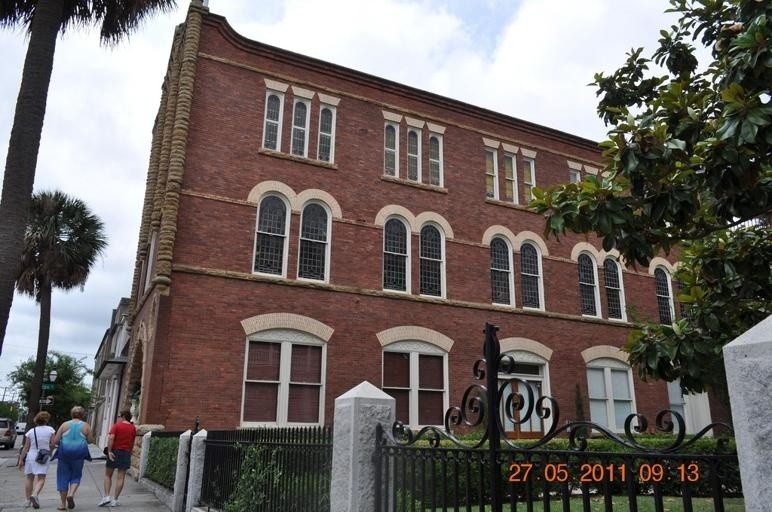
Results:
[35,448,51,463]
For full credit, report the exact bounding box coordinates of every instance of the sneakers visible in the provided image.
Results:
[58,505,66,511]
[111,499,121,507]
[98,496,111,508]
[30,494,40,508]
[23,501,31,508]
[67,495,75,509]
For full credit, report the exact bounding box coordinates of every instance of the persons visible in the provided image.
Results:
[98,410,137,507]
[54,406,94,510]
[19,410,57,509]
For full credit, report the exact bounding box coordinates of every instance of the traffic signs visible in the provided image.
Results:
[42,384,54,390]
[39,397,55,406]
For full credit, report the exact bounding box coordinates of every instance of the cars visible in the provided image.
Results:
[14,421,27,435]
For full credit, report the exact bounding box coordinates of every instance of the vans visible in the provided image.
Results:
[0,417,18,451]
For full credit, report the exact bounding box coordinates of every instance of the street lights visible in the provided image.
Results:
[46,369,58,413]
